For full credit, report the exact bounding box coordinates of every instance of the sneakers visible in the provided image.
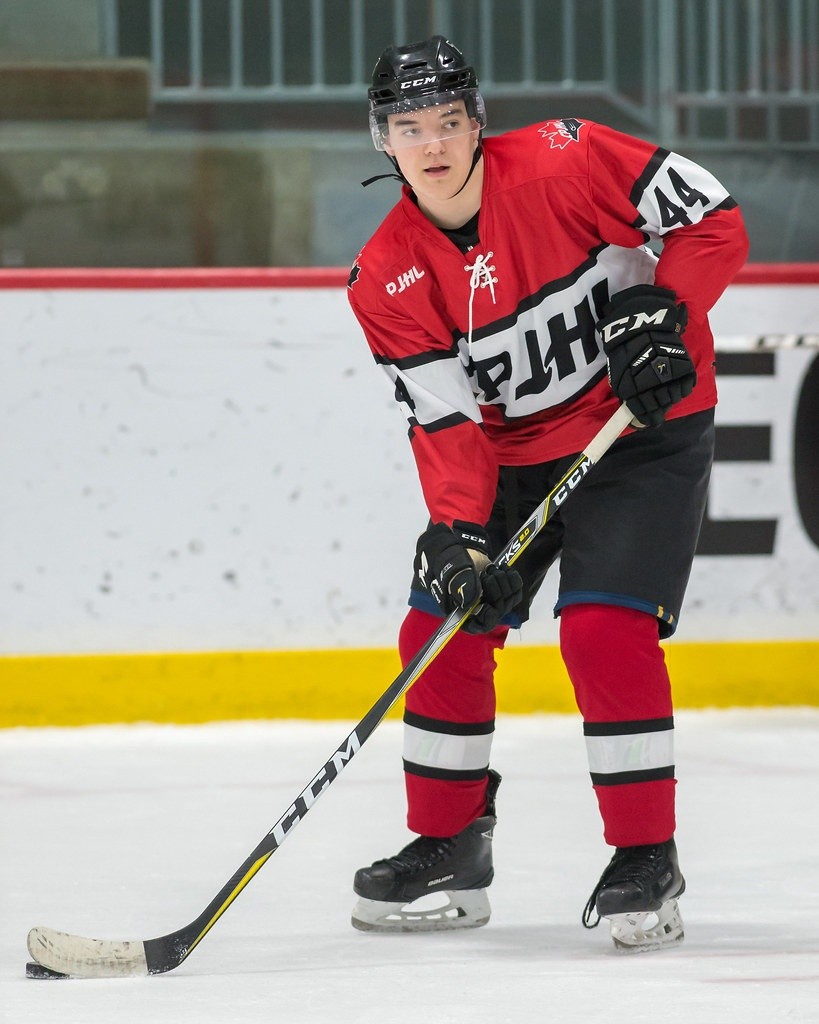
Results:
[350,768,503,934]
[582,837,687,955]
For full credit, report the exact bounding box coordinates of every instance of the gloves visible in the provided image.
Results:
[595,284,697,433]
[413,519,523,635]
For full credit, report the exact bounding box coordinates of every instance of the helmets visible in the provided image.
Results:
[360,35,487,200]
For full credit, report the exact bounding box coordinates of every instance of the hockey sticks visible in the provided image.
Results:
[26,396,632,980]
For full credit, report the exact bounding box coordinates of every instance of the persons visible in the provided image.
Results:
[345,36,749,951]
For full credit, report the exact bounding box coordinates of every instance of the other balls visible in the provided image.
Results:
[25,963,69,980]
